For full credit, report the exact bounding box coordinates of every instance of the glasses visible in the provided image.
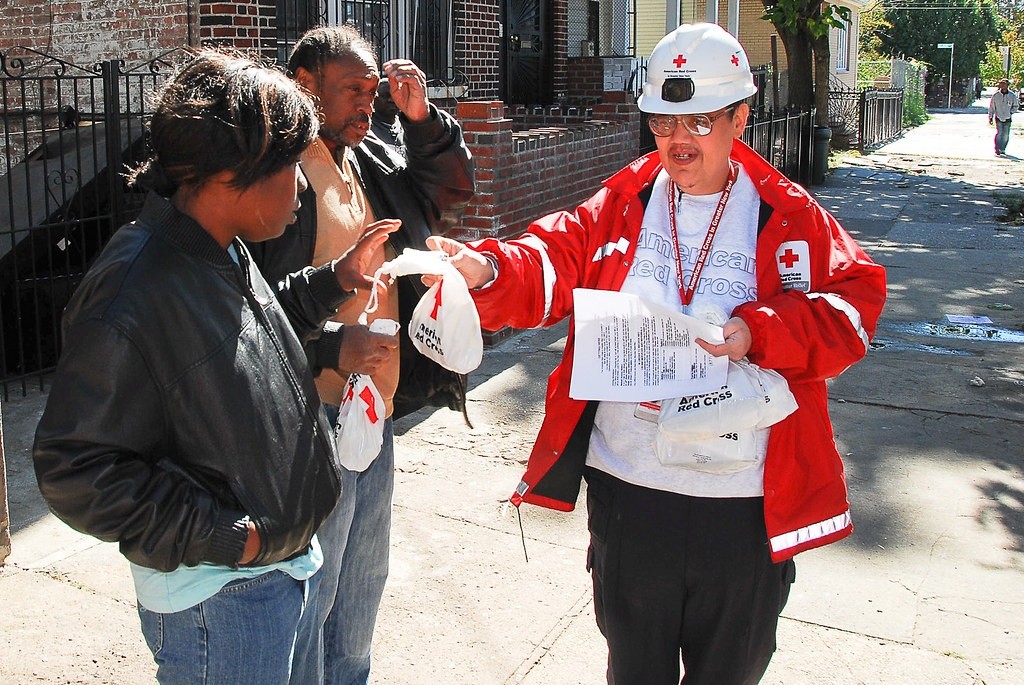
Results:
[645,106,733,138]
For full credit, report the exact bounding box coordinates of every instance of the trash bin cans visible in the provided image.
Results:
[799,125,831,184]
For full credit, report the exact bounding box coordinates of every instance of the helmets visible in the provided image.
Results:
[636,22,758,115]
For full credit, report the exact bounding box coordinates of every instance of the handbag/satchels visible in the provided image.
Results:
[365,247,483,374]
[335,319,401,472]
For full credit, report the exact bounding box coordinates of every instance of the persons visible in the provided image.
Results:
[32,52,402,685]
[421,22,885,685]
[976,79,1019,156]
[237,27,475,685]
